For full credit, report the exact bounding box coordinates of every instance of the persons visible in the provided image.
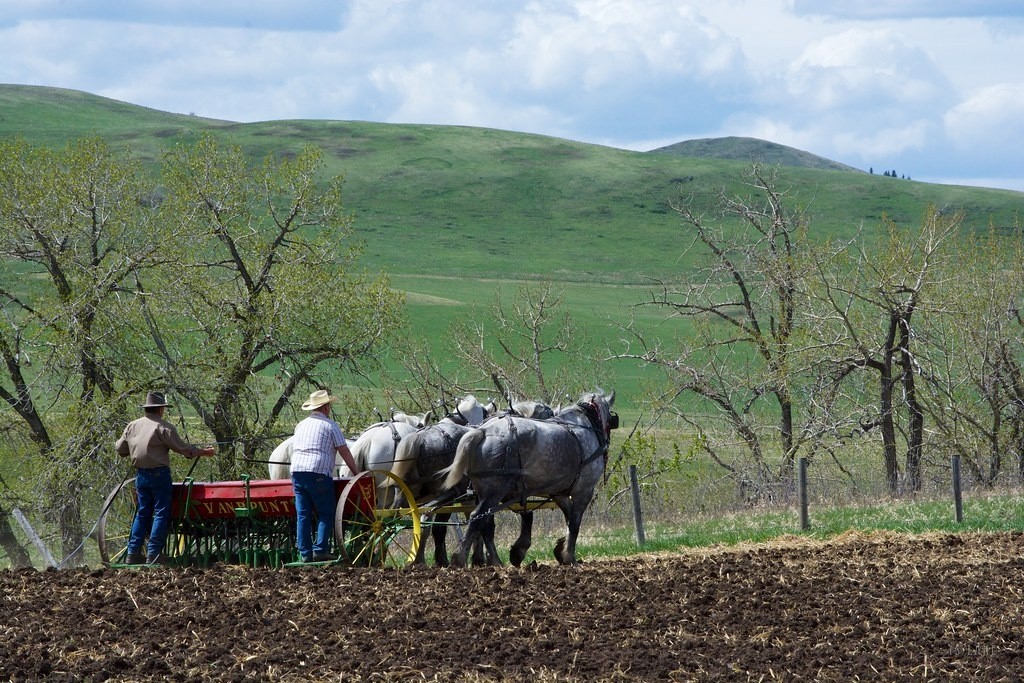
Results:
[115,391,215,564]
[290,391,368,562]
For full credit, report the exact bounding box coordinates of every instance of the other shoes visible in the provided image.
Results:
[301,553,312,562]
[313,553,337,560]
[125,553,147,565]
[146,554,176,563]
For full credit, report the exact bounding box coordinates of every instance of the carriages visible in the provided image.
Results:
[94,383,618,571]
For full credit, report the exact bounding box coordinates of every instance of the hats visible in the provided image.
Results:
[300,389,338,411]
[142,391,175,408]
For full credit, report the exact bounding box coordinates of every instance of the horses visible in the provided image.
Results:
[269,390,615,572]
